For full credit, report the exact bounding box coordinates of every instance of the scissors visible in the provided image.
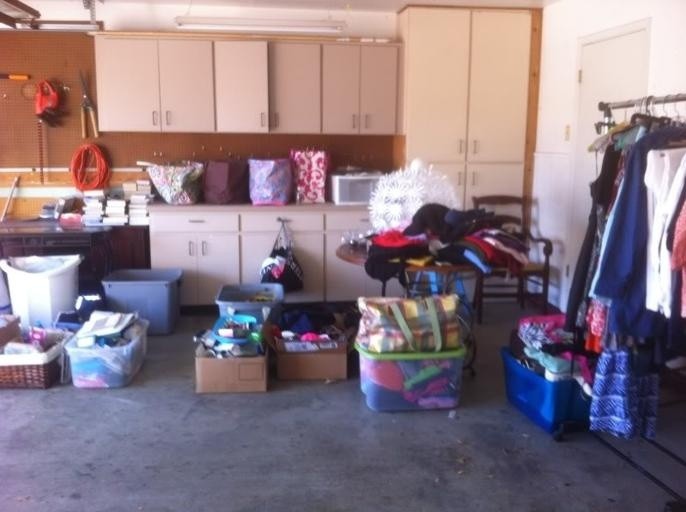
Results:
[78,69,100,139]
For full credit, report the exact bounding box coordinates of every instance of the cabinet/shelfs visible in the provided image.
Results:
[212,30,322,135]
[322,36,405,135]
[240,202,325,309]
[86,30,216,134]
[147,205,240,314]
[325,204,409,302]
[407,164,526,231]
[392,7,532,164]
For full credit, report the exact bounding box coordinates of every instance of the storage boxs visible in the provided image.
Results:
[497,345,593,436]
[276,341,349,380]
[100,267,181,330]
[353,340,467,413]
[215,283,284,333]
[65,318,151,386]
[194,341,271,393]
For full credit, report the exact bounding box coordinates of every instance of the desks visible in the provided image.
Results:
[337,241,481,320]
[3,221,116,288]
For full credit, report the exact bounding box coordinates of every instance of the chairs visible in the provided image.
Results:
[472,195,553,316]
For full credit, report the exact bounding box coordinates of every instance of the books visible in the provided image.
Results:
[122,178,137,199]
[137,178,152,194]
[129,193,150,227]
[105,199,129,226]
[81,189,104,227]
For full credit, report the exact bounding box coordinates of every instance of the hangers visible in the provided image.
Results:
[585,93,685,152]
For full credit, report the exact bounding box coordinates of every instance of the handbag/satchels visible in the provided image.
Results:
[353,292,463,352]
